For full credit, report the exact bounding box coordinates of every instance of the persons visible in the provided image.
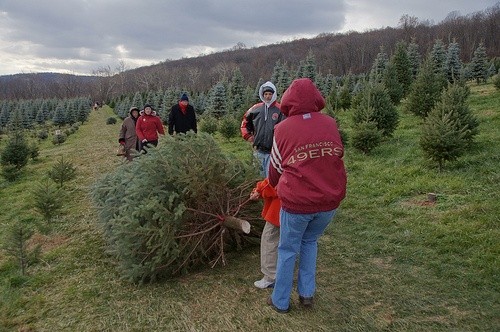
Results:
[241,81,286,178]
[267,78,347,313]
[94,101,166,161]
[169,94,197,134]
[250,180,282,289]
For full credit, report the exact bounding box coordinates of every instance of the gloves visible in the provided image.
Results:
[120,140,125,146]
[142,139,147,143]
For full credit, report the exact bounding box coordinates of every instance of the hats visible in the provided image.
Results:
[262,87,274,97]
[181,93,188,102]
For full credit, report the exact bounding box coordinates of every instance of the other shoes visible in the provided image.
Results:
[267,295,289,313]
[299,295,313,305]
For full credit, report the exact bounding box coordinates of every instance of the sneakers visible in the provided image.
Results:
[254,277,273,288]
